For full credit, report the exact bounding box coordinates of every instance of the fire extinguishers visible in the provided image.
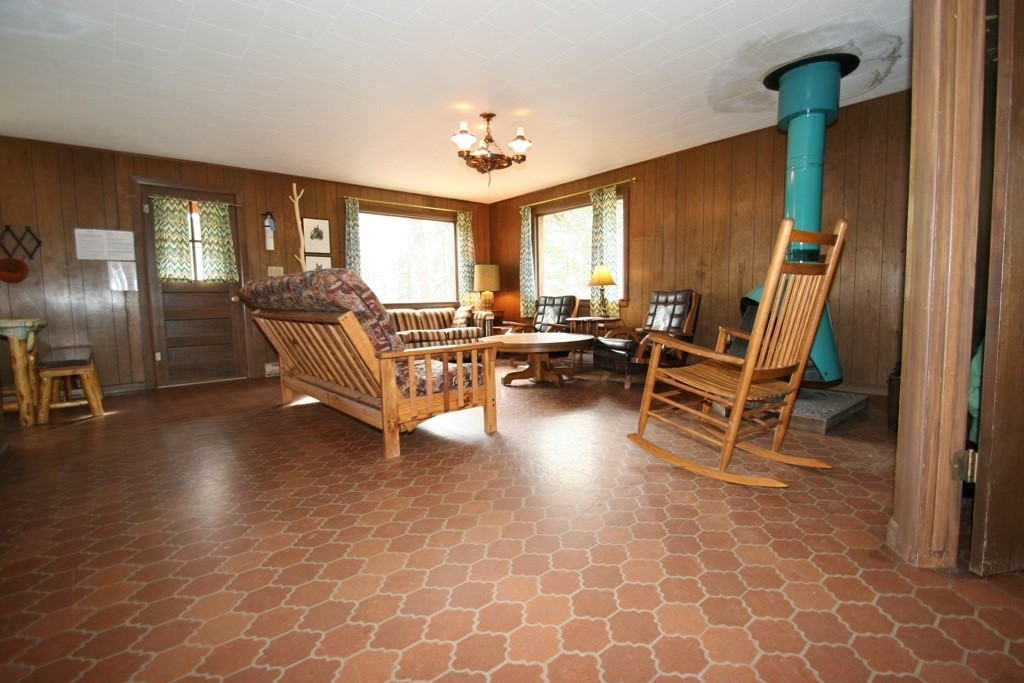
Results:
[261,211,276,250]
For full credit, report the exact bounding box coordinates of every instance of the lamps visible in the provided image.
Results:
[474,264,500,309]
[589,266,616,318]
[451,111,531,173]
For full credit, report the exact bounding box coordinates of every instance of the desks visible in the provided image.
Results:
[478,334,595,388]
[565,317,621,368]
[491,309,504,326]
[0,319,47,426]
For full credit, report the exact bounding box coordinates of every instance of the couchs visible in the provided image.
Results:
[383,305,495,362]
[242,266,504,457]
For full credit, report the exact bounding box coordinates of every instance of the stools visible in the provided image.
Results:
[36,345,103,424]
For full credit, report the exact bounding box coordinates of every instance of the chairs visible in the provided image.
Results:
[593,289,702,389]
[628,219,850,487]
[500,295,579,357]
